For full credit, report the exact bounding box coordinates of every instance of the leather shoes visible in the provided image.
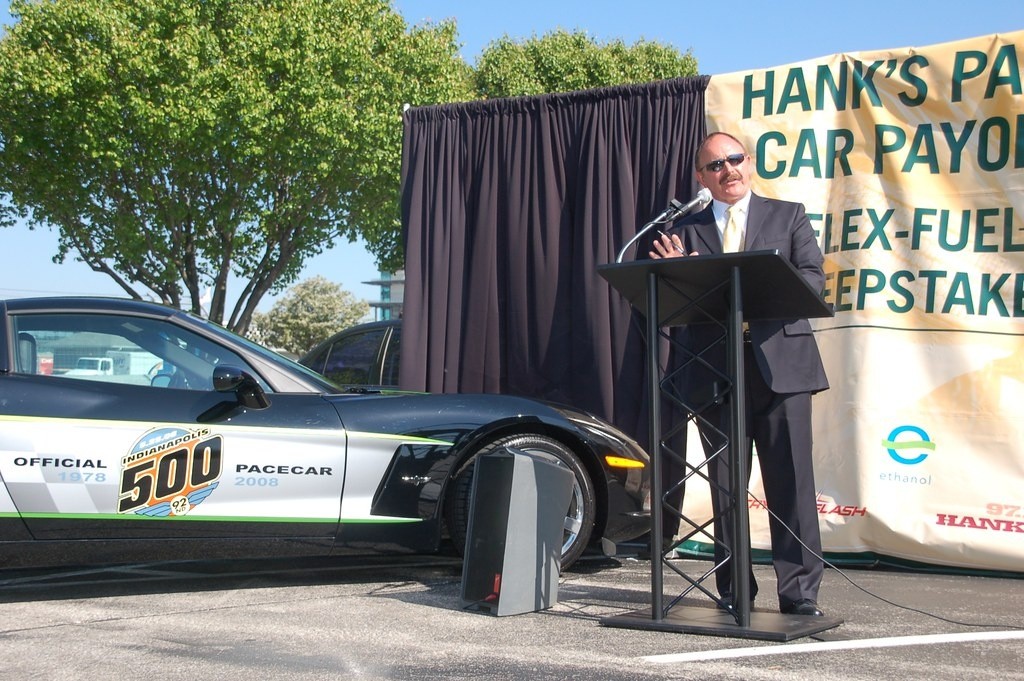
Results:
[780,599,823,617]
[716,597,754,610]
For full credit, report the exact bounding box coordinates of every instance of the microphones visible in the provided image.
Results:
[666,187,712,223]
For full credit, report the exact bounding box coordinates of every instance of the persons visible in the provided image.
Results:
[648,132,829,615]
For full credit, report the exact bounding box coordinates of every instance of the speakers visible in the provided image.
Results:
[460,446,576,617]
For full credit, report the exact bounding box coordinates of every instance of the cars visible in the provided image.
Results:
[294,318,402,392]
[1,296,653,576]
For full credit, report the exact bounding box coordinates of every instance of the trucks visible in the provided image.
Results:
[63,350,177,385]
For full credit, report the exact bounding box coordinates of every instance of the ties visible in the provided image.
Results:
[722,206,742,254]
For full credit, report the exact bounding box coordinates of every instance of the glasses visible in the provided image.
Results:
[699,153,748,172]
